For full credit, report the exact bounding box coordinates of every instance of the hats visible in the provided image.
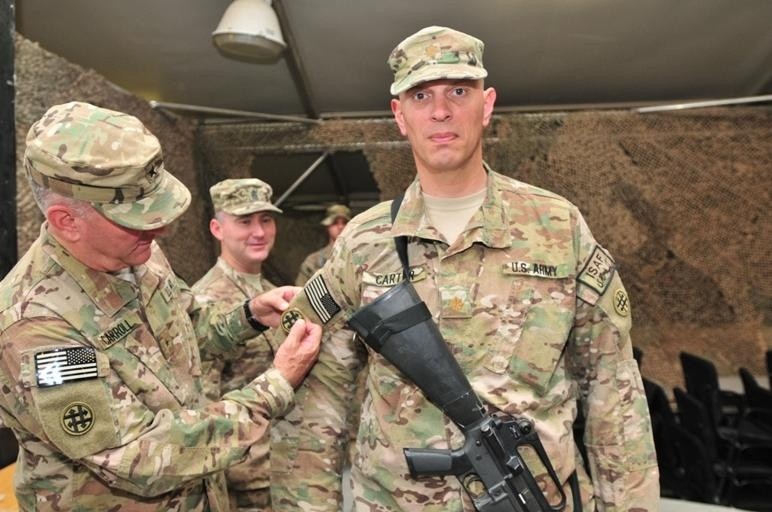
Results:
[209,178,284,216]
[388,25,488,96]
[321,205,351,226]
[24,101,193,231]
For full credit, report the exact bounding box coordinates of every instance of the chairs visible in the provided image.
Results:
[631,348,770,505]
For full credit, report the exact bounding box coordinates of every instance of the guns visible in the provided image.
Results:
[346,278,567,511]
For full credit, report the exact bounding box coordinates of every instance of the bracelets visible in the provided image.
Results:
[241,299,270,333]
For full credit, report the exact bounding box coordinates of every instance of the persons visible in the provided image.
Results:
[290,203,353,287]
[180,175,286,511]
[0,97,325,510]
[261,22,665,510]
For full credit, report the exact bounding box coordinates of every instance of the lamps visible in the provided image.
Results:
[209,0,289,66]
